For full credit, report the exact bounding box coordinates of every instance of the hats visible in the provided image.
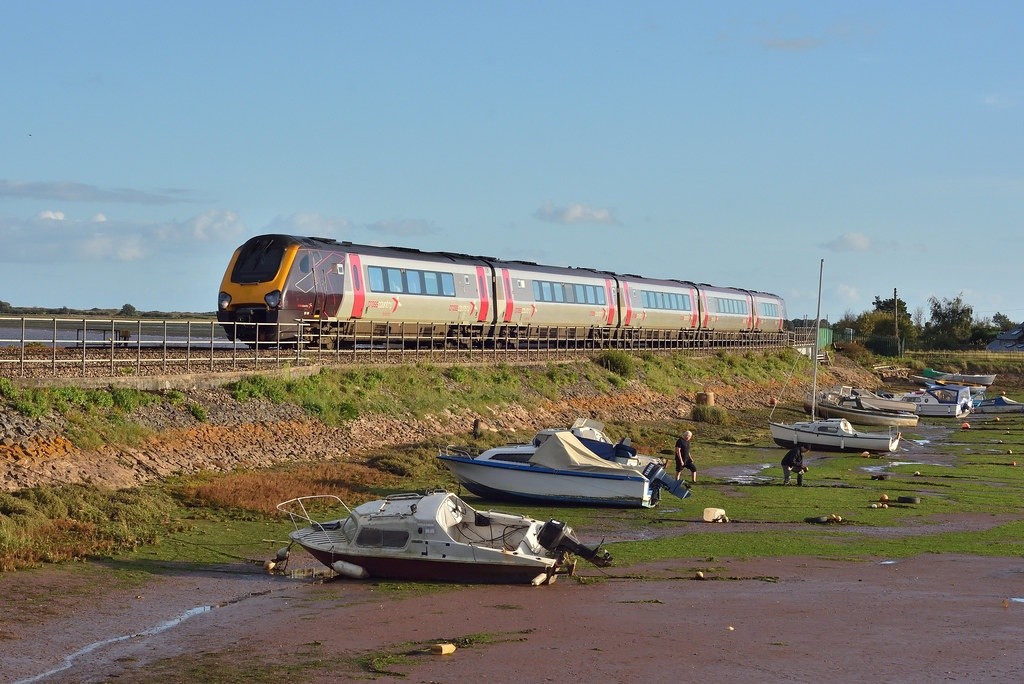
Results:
[804,444,811,450]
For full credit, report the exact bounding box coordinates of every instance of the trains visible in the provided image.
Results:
[217,234,784,349]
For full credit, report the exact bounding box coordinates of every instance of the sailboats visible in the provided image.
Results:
[769,258,901,452]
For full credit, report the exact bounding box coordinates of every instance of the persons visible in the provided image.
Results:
[781,445,810,486]
[675,431,697,483]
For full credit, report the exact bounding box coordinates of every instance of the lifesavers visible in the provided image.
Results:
[898,496,920,504]
[806,516,828,523]
[872,474,890,481]
[940,391,953,401]
[616,444,637,456]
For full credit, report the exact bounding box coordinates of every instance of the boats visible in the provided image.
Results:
[436,417,692,509]
[923,367,996,388]
[804,385,919,426]
[971,396,1024,413]
[935,380,981,394]
[821,385,917,413]
[276,488,614,585]
[877,381,973,419]
